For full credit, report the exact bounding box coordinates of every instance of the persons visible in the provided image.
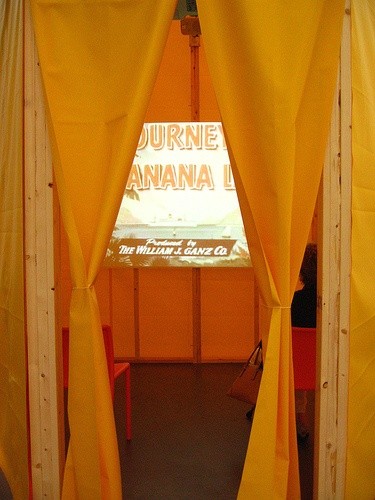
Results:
[244,240,318,443]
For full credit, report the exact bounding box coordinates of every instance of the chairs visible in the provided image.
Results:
[63,324,132,444]
[291,327,316,390]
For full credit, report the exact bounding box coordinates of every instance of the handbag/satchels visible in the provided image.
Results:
[226,341,263,405]
